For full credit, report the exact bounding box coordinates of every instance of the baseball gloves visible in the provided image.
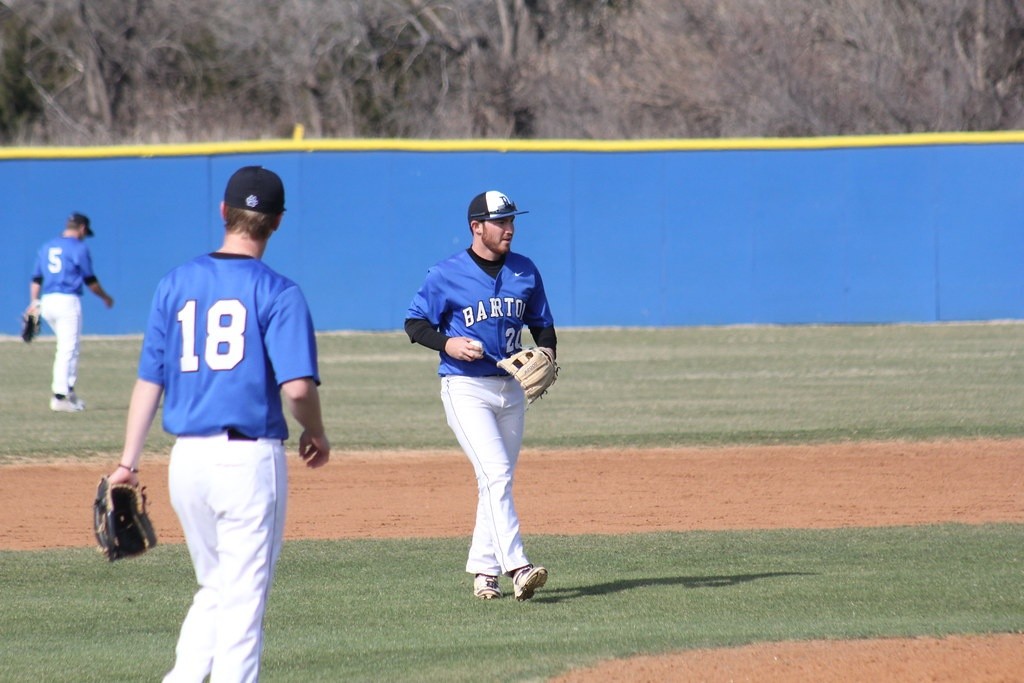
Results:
[495,346,561,404]
[93,472,158,563]
[21,314,42,342]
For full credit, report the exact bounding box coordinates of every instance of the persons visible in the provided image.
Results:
[405,190,558,601]
[107,167,331,683]
[26,213,113,413]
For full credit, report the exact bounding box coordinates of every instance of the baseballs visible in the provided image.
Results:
[469,341,483,355]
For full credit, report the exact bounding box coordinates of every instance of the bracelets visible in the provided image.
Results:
[118,463,138,473]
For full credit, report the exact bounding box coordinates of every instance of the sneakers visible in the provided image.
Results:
[513,566,548,602]
[473,574,503,599]
[50,391,86,413]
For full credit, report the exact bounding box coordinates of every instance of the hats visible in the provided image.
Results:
[468,190,529,225]
[224,166,287,215]
[67,213,94,237]
